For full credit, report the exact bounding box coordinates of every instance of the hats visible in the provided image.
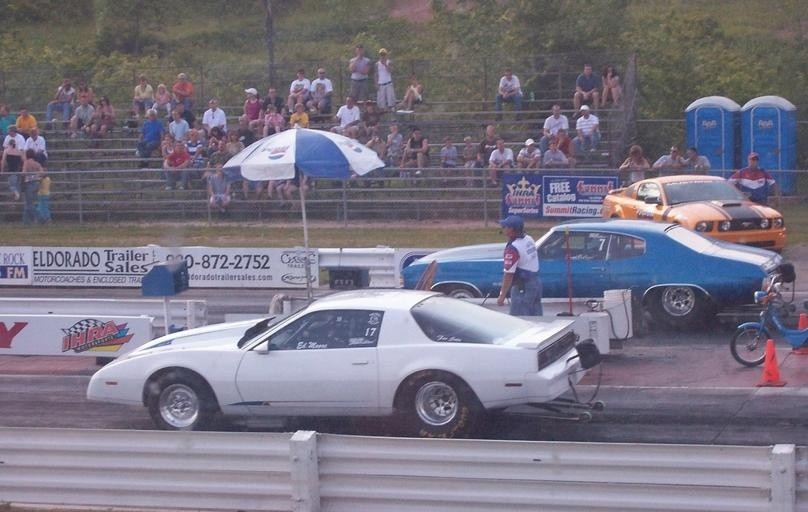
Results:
[378,48,387,54]
[579,104,590,113]
[524,138,535,147]
[244,87,259,96]
[748,153,759,159]
[494,214,524,230]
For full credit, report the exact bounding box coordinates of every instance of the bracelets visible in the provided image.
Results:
[499,290,505,296]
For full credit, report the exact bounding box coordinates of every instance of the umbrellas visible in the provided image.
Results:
[220,121,386,299]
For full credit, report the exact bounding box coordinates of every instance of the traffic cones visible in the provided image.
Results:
[788,313,808,355]
[755,338,787,387]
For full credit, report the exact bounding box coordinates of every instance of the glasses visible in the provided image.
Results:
[752,158,760,161]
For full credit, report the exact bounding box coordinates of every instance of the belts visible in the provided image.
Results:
[351,78,366,81]
[379,81,391,86]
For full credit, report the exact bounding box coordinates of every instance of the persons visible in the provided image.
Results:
[0,106,50,225]
[132,73,247,198]
[348,44,424,114]
[651,145,683,177]
[494,68,523,121]
[572,64,621,121]
[683,147,711,174]
[727,150,780,208]
[330,95,429,179]
[439,125,569,186]
[46,78,114,140]
[491,217,544,316]
[242,67,333,140]
[540,103,601,154]
[619,145,651,185]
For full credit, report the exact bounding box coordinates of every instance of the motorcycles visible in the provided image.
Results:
[730,263,807,367]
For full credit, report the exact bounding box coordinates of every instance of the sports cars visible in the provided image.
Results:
[401,217,786,329]
[600,174,786,255]
[85,287,597,439]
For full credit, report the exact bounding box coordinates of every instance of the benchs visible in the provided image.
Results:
[0,89,627,216]
